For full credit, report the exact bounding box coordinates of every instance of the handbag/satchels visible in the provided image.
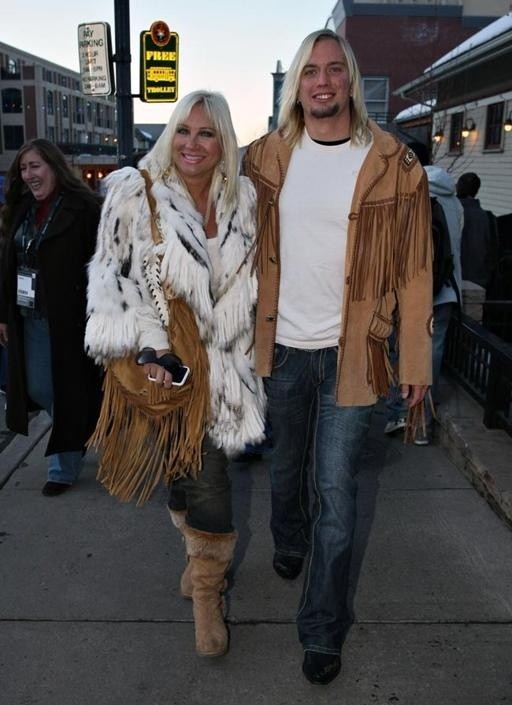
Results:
[429,196,454,297]
[108,297,194,419]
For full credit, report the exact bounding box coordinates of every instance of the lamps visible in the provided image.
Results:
[433,125,444,141]
[460,117,475,138]
[502,110,512,132]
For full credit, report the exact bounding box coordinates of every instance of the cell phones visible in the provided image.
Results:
[149,367,192,385]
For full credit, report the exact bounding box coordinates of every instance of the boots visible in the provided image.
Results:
[179,524,239,658]
[166,503,193,599]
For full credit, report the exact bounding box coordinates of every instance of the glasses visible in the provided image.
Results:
[135,347,183,375]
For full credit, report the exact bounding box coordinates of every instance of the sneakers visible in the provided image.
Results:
[412,426,432,445]
[303,651,340,685]
[42,481,70,497]
[273,551,304,579]
[384,418,412,437]
